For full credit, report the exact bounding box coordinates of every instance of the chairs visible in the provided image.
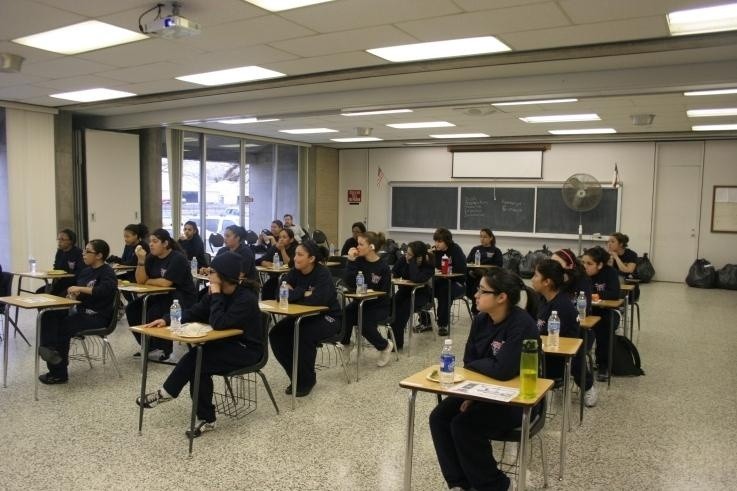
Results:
[418,283,438,341]
[484,397,547,490]
[453,291,475,328]
[359,285,400,363]
[312,288,355,384]
[128,321,245,452]
[203,253,213,266]
[567,345,594,394]
[211,312,279,418]
[68,292,124,379]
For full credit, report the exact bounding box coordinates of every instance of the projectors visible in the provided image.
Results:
[139,12,202,41]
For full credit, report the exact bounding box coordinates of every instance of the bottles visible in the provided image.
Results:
[548,310,559,350]
[28,255,37,274]
[442,256,448,274]
[171,300,181,333]
[440,339,454,388]
[191,257,197,278]
[356,271,364,294]
[330,243,334,256]
[577,292,586,321]
[280,281,288,311]
[274,253,280,270]
[475,250,481,267]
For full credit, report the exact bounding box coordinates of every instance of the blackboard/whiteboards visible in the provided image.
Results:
[386,181,622,241]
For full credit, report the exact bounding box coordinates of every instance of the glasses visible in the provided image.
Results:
[83,248,98,254]
[210,268,218,273]
[477,287,500,294]
[56,238,71,242]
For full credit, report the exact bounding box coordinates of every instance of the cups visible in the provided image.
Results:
[441,253,448,274]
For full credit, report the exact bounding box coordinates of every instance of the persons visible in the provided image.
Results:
[340,222,367,257]
[34,230,85,314]
[385,240,435,352]
[603,233,640,314]
[526,258,581,381]
[429,269,545,491]
[411,227,467,336]
[260,220,283,246]
[282,214,307,243]
[123,228,194,361]
[549,249,597,409]
[176,220,208,274]
[102,223,151,322]
[257,229,300,300]
[577,245,621,381]
[462,228,502,321]
[269,241,342,398]
[137,253,267,438]
[35,240,117,384]
[212,224,261,290]
[342,231,393,367]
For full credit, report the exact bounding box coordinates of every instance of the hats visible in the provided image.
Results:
[211,251,242,281]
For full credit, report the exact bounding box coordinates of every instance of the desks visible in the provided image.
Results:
[1,290,83,402]
[107,259,135,282]
[192,268,216,291]
[117,278,177,356]
[433,270,467,338]
[398,361,554,487]
[8,268,77,340]
[340,284,387,382]
[255,261,296,290]
[619,280,639,339]
[577,311,602,332]
[255,298,331,411]
[465,261,499,275]
[588,296,628,389]
[388,273,428,358]
[533,332,585,431]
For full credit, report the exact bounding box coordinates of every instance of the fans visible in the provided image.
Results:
[562,172,606,256]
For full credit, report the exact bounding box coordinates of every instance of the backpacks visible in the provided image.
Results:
[596,334,646,377]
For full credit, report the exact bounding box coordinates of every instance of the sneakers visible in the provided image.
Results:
[341,341,354,366]
[450,487,462,490]
[186,420,216,437]
[148,348,173,362]
[471,311,480,320]
[133,346,141,358]
[136,390,175,408]
[39,347,62,365]
[584,385,598,406]
[508,479,516,491]
[413,324,432,332]
[377,339,393,367]
[596,365,608,380]
[39,371,69,384]
[438,325,448,336]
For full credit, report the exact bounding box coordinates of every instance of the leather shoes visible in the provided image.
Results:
[296,373,317,396]
[286,383,292,394]
